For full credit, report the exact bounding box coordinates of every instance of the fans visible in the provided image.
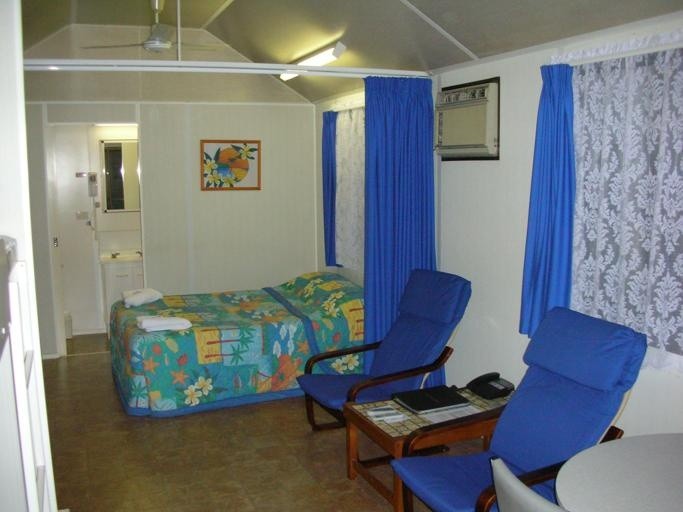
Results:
[80,1,226,54]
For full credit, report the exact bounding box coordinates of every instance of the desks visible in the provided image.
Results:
[552,432,683,512]
[340,388,517,510]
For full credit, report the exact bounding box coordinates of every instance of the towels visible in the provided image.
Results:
[122,286,192,332]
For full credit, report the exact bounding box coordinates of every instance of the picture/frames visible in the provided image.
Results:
[199,139,262,192]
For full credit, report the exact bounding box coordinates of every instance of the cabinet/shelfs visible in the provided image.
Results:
[102,262,143,332]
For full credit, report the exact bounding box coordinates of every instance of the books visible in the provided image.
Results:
[393,385,471,414]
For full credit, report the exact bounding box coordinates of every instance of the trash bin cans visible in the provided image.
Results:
[64,311,72,339]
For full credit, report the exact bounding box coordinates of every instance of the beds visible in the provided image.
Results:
[105,272,364,417]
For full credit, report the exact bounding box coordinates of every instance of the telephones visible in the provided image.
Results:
[466,372,515,400]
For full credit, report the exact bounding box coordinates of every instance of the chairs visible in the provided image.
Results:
[389,305,650,510]
[297,266,474,432]
[488,456,566,511]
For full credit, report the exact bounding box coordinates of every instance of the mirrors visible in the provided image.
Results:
[98,139,140,213]
